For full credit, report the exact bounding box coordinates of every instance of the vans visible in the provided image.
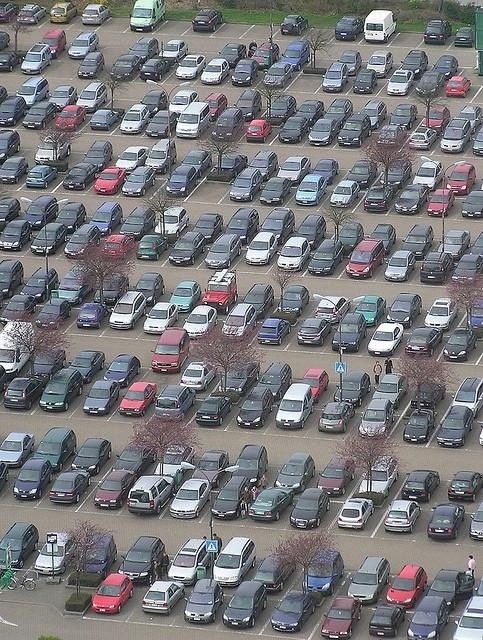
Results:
[212,537,257,585]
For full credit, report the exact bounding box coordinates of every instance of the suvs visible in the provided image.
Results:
[0,522,39,569]
[184,578,224,624]
[167,537,211,586]
[302,548,347,595]
[117,535,167,584]
[74,532,117,578]
[222,581,268,629]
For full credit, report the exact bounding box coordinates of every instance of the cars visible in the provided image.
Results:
[320,556,483,639]
[35,531,77,576]
[91,572,133,614]
[252,553,297,592]
[140,580,185,616]
[0,0,482,541]
[270,594,316,632]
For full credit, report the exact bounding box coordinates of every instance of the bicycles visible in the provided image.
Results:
[5,571,37,592]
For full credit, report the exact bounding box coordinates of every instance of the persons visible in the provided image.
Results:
[240,474,266,518]
[468,555,476,575]
[384,356,393,374]
[373,361,382,385]
[213,534,222,560]
[153,550,169,580]
[466,568,472,576]
